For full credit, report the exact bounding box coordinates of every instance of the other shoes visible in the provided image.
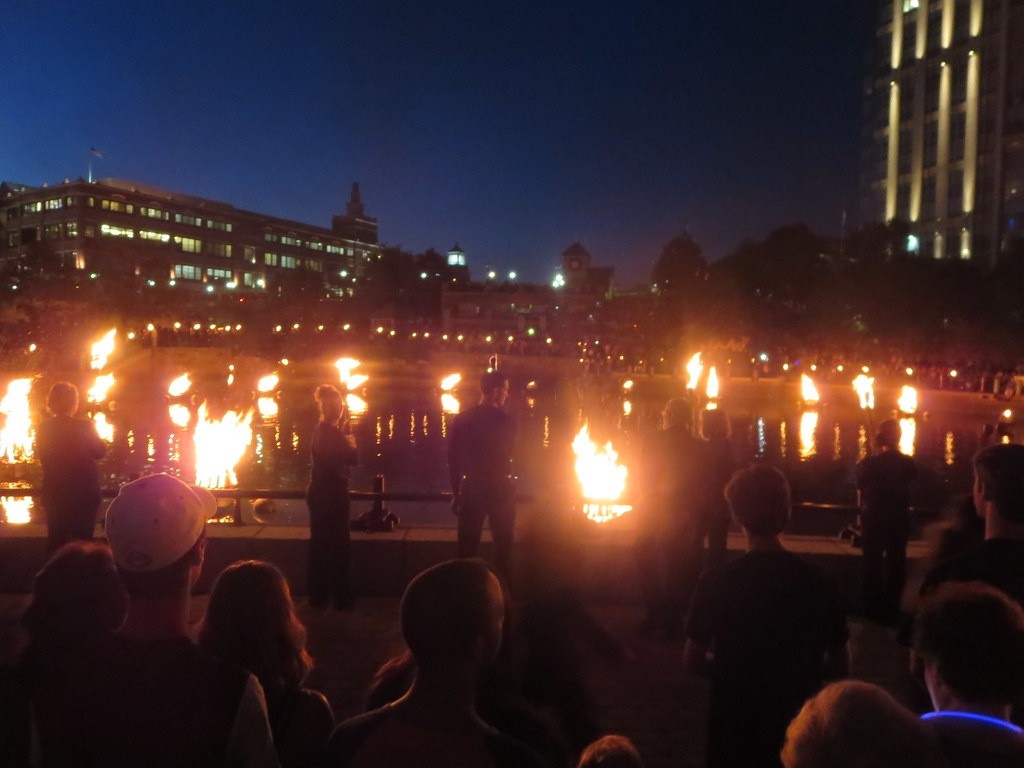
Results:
[311,594,338,608]
[635,617,657,634]
[658,623,686,643]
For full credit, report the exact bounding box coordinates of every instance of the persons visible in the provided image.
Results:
[637,399,713,569]
[447,372,516,591]
[699,410,732,570]
[851,419,917,614]
[37,383,103,561]
[306,386,361,606]
[0,442,1024,768]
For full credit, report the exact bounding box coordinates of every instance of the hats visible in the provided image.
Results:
[104,474,218,572]
